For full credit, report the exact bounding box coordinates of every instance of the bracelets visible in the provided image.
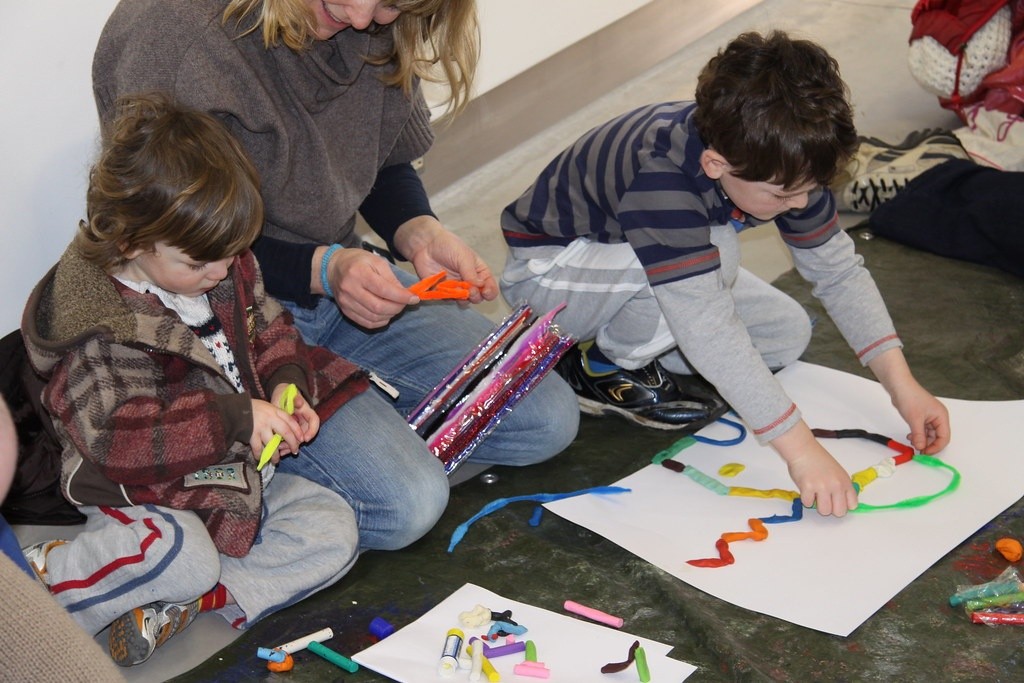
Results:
[321,243,343,298]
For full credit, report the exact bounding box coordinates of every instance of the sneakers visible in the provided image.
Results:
[110,600,198,667]
[553,337,730,431]
[842,126,971,213]
[21,539,72,592]
[845,127,932,178]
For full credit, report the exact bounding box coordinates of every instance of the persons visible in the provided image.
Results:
[91,0,579,551]
[828,0,1024,270]
[20,90,370,668]
[498,30,951,518]
